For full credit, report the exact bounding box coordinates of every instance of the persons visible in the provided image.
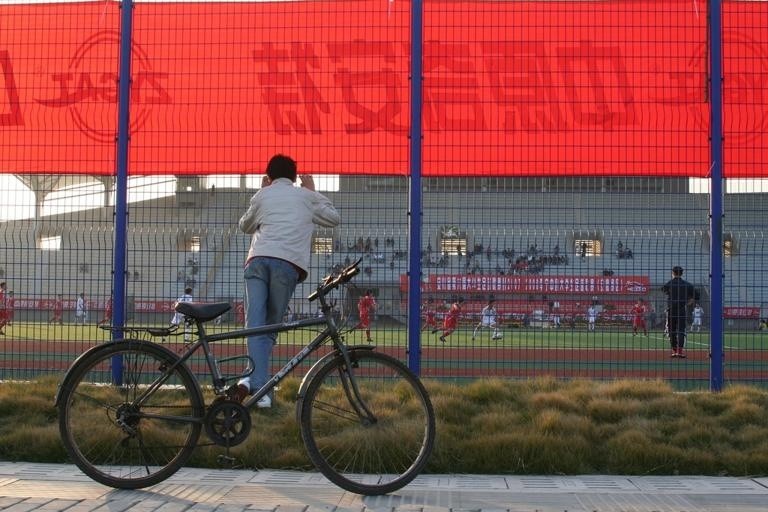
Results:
[660,266,701,359]
[456,242,569,277]
[98,289,113,328]
[0,281,11,336]
[419,294,706,342]
[581,242,587,261]
[73,293,87,327]
[284,304,293,324]
[616,241,632,258]
[604,268,614,275]
[327,237,407,275]
[170,287,194,342]
[228,154,343,410]
[134,270,139,280]
[349,289,379,342]
[48,294,65,326]
[176,257,201,289]
[421,245,449,268]
[5,291,16,327]
[234,301,245,328]
[1,267,4,279]
[756,318,768,333]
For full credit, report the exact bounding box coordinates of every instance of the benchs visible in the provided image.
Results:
[1,191,767,321]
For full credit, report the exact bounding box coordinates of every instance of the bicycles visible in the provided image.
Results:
[53,256,437,497]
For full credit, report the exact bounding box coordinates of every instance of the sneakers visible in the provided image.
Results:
[229,377,250,404]
[256,394,271,410]
[671,353,685,358]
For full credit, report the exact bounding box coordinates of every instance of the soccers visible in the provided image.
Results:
[497,332,502,339]
